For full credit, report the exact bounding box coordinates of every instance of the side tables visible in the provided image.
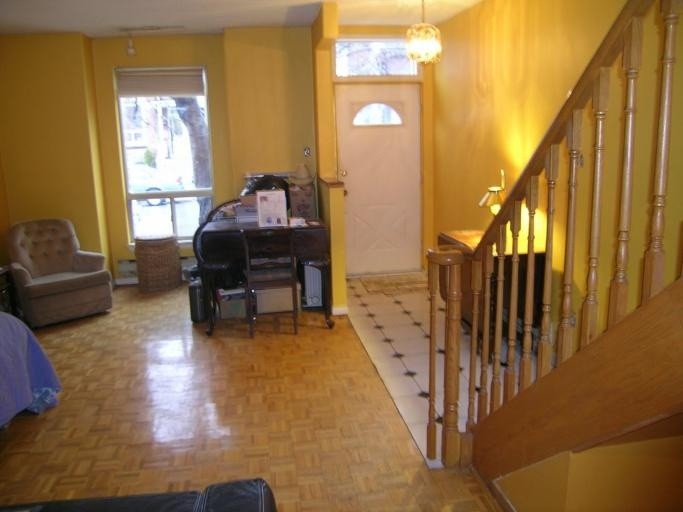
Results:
[0,264,18,319]
[134,233,182,292]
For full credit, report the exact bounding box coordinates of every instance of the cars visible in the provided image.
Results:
[127,163,185,206]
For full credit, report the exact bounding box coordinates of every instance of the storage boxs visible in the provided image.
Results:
[288,184,316,219]
[254,280,301,314]
[217,298,247,320]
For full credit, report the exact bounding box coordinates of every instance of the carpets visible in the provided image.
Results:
[346,273,556,470]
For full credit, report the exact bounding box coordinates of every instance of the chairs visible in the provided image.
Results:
[239,227,299,339]
[6,218,113,328]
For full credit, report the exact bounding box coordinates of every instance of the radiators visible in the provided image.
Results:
[302,264,323,308]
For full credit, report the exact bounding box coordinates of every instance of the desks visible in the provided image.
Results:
[199,218,334,336]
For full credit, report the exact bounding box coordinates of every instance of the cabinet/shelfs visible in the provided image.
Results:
[437,232,546,365]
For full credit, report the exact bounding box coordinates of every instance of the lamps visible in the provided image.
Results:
[404,0,442,65]
